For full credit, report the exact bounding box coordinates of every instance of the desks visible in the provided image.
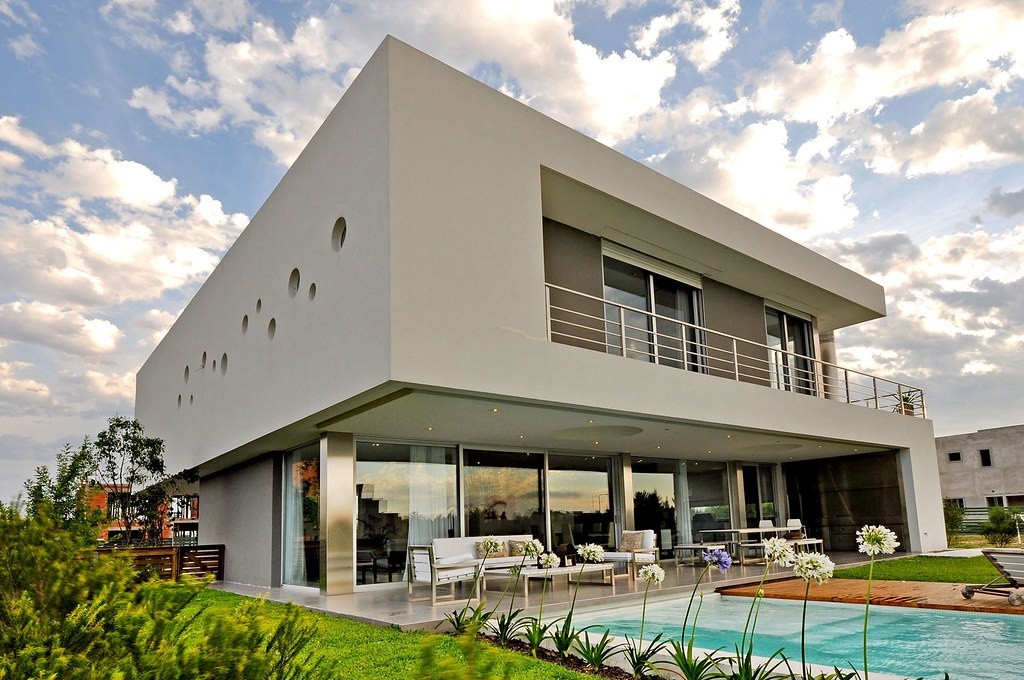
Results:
[482,563,615,597]
[555,532,609,543]
[699,527,802,568]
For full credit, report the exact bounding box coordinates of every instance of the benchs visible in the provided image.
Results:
[728,540,757,555]
[739,541,796,567]
[432,534,555,582]
[674,545,726,568]
[960,549,1024,607]
[677,542,730,564]
[788,539,824,555]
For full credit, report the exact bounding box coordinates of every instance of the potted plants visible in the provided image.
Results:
[889,388,926,417]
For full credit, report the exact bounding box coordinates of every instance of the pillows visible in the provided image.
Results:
[475,541,509,559]
[508,539,529,556]
[619,531,644,552]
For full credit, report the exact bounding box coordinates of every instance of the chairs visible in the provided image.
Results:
[593,523,601,532]
[561,523,586,562]
[601,529,660,580]
[575,523,583,532]
[356,549,407,583]
[786,518,810,554]
[407,544,485,606]
[759,519,778,555]
[531,525,544,544]
[600,522,615,548]
